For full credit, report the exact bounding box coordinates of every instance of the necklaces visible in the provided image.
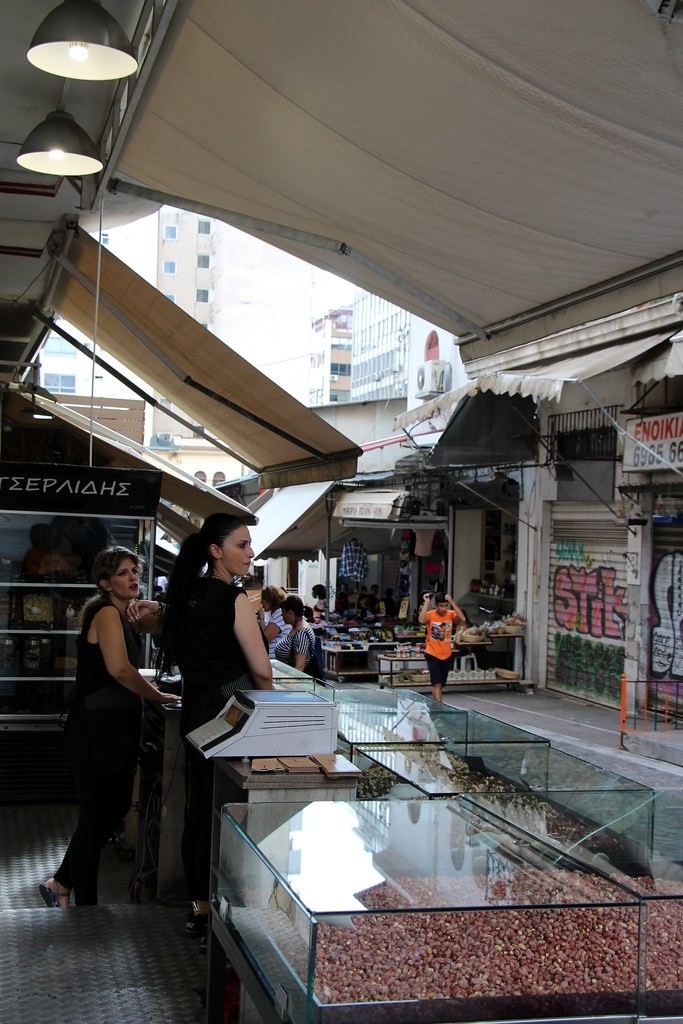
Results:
[204,573,228,584]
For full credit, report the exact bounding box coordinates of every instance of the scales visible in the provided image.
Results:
[185,689,339,761]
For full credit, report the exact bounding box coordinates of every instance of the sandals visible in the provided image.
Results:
[39,878,70,907]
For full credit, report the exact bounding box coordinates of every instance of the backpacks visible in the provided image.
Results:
[289,629,326,687]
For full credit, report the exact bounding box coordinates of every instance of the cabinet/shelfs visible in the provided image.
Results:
[377,635,529,689]
[0,460,163,807]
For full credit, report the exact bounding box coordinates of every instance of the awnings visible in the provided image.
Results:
[55,223,364,490]
[246,379,538,561]
[106,0,683,404]
[0,380,254,577]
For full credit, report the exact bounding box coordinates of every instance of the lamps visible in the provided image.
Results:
[14,104,105,177]
[26,0,139,81]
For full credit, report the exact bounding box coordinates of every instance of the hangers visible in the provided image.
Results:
[347,532,359,545]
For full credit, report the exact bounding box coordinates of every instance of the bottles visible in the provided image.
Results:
[655,494,663,514]
[470,579,504,597]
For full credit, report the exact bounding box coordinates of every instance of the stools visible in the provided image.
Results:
[453,653,478,671]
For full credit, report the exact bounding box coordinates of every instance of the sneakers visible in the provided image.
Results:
[184,911,208,938]
[199,924,223,955]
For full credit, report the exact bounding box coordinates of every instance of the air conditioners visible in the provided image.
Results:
[415,360,446,398]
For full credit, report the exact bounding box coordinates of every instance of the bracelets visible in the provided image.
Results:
[258,617,265,619]
[152,600,166,616]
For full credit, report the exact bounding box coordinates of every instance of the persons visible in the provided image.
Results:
[334,582,399,622]
[417,591,466,703]
[38,544,182,907]
[452,609,475,658]
[18,514,121,583]
[256,585,294,659]
[311,584,326,623]
[273,595,316,674]
[149,512,275,954]
